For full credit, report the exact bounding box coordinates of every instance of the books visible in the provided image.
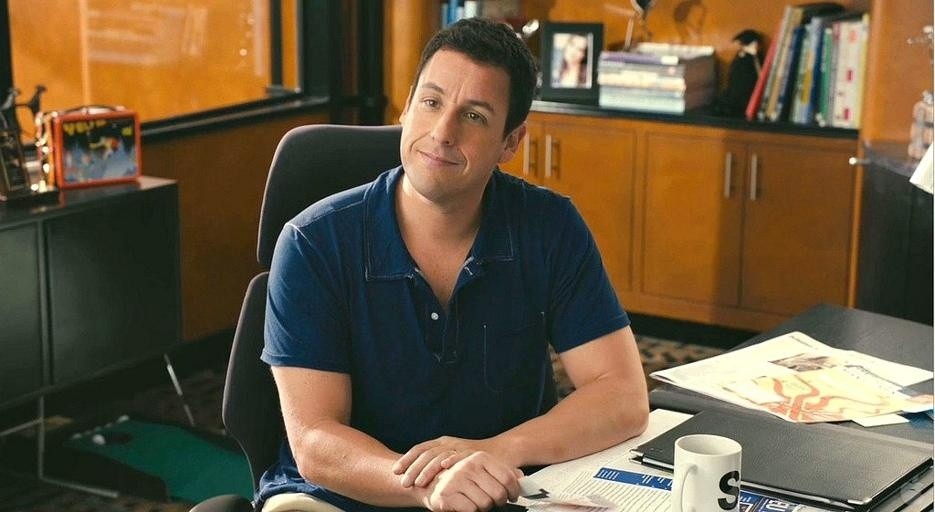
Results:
[743,2,872,130]
[593,41,719,116]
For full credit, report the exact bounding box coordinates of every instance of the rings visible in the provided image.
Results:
[446,449,458,454]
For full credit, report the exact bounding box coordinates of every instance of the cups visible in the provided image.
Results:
[670,433,744,512]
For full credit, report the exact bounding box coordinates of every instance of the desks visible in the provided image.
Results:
[481,302,934,512]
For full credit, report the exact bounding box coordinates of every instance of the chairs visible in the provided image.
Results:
[183,123,404,511]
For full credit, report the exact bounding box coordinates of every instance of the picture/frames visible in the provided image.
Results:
[538,20,603,104]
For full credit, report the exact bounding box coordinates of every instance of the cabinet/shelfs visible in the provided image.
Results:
[496,102,638,331]
[1,164,182,480]
[636,109,862,332]
[854,139,934,325]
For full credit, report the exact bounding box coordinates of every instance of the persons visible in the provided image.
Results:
[557,33,587,88]
[254,14,650,511]
